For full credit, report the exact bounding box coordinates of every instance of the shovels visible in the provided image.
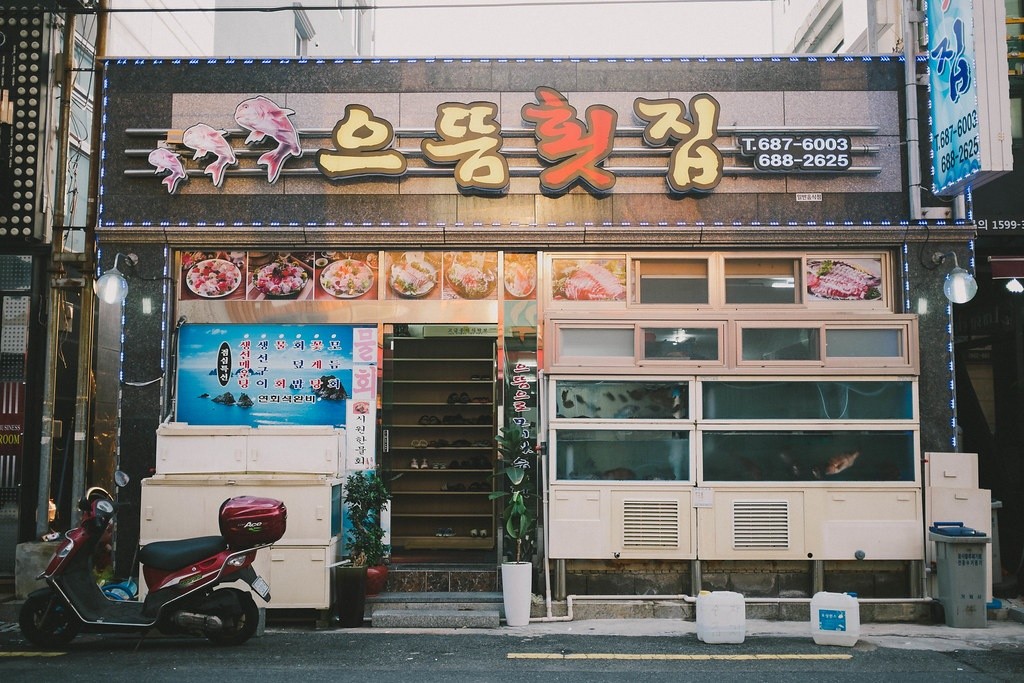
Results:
[112,540,141,600]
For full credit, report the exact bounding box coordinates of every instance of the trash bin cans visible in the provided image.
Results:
[929,522,990,628]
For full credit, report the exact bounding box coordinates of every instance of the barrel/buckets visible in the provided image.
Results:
[810,591,860,647]
[695,590,745,643]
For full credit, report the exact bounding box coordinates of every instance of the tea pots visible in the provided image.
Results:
[297,254,309,265]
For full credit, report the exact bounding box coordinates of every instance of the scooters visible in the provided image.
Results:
[18,470,288,652]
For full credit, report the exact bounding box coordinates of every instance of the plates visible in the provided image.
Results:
[445,262,496,299]
[186,259,242,298]
[504,261,537,297]
[366,259,378,270]
[808,258,882,301]
[252,262,308,296]
[388,261,436,298]
[320,259,374,298]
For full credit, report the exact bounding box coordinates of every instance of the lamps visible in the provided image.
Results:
[96,252,141,304]
[932,251,980,303]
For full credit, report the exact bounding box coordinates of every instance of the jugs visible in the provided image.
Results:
[231,251,246,264]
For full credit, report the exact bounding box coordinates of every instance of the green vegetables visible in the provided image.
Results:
[393,261,488,298]
[816,259,834,275]
[864,286,882,300]
[325,260,364,296]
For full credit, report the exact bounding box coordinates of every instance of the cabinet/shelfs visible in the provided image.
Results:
[380,335,496,551]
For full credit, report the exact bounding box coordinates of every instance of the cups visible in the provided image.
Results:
[443,252,453,262]
[316,258,328,266]
[400,252,425,262]
[237,260,244,267]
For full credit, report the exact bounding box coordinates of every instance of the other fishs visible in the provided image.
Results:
[614,404,640,418]
[664,396,680,413]
[809,465,823,480]
[642,471,677,480]
[234,95,301,184]
[183,123,238,187]
[618,394,627,403]
[569,458,636,481]
[808,260,882,286]
[650,405,661,412]
[561,389,585,408]
[607,392,615,401]
[779,452,802,475]
[825,448,860,475]
[148,147,185,193]
[626,389,642,400]
[806,270,882,300]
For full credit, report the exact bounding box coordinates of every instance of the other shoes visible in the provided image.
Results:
[442,415,493,425]
[428,439,492,447]
[440,479,492,492]
[481,375,490,381]
[417,415,431,425]
[432,455,492,469]
[410,440,420,447]
[443,528,457,536]
[435,528,445,536]
[410,457,419,469]
[419,440,428,448]
[421,458,428,468]
[480,529,487,537]
[471,374,480,381]
[470,528,478,537]
[429,416,441,425]
[471,395,489,402]
[447,393,459,403]
[459,392,471,402]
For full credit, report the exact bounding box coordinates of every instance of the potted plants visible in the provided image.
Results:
[332,474,388,629]
[489,423,534,629]
